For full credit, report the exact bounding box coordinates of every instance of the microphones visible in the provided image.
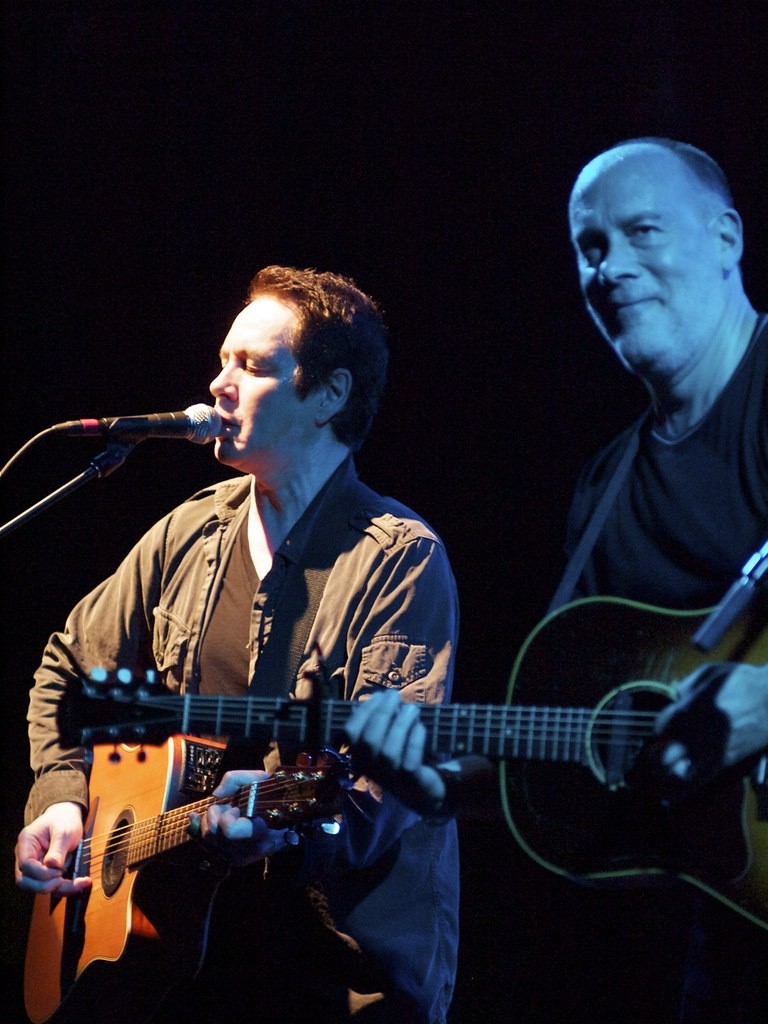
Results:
[66,402,224,446]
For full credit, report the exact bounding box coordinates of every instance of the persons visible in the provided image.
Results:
[342,138,768,1024]
[16,265,461,1023]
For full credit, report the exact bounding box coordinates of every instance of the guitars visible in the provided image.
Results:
[19,732,348,1023]
[53,584,768,935]
